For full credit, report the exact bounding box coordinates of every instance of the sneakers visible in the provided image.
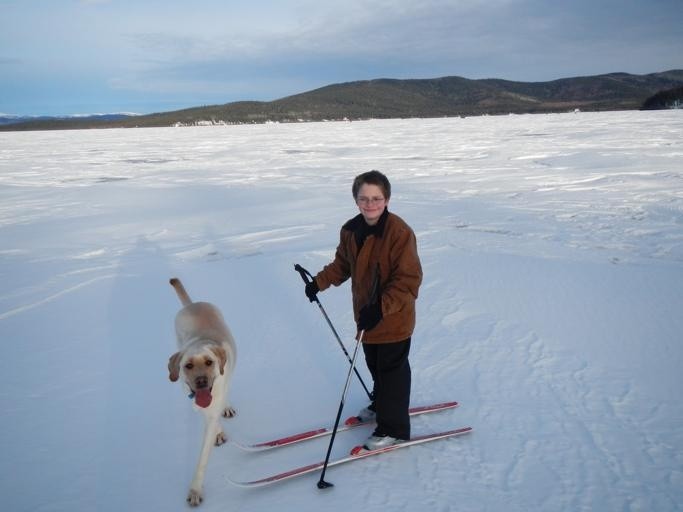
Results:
[359,407,376,421]
[364,432,406,450]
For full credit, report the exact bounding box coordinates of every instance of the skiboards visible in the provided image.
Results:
[227,401,472,488]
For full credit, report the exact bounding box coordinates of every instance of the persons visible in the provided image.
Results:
[305,170,423,450]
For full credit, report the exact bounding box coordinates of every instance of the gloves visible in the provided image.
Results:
[358,303,383,331]
[305,282,321,303]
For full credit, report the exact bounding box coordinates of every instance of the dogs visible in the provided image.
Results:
[166,276,238,509]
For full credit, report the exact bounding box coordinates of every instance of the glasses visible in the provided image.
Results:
[356,197,386,204]
